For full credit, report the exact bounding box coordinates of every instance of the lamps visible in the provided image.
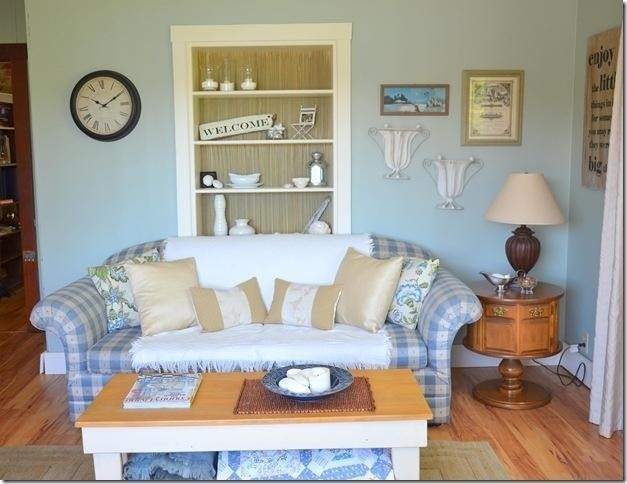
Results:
[485,172,568,289]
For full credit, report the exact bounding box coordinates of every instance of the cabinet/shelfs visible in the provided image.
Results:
[169,23,354,236]
[0,43,24,297]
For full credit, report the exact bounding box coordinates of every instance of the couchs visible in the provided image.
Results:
[28,233,484,429]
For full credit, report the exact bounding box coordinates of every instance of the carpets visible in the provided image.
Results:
[0,442,513,483]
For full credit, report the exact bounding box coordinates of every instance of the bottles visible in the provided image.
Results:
[307,151,328,189]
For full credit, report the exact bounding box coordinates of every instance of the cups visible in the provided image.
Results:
[519,273,537,296]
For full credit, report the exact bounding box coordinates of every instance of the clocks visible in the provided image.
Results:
[69,69,141,144]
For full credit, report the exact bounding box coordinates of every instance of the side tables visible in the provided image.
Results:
[461,277,564,410]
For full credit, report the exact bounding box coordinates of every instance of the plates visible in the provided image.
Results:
[227,181,264,188]
[260,363,355,402]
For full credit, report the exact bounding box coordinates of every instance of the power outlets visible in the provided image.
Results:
[581,332,588,355]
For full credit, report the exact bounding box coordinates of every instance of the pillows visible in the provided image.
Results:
[385,254,441,333]
[264,280,342,333]
[330,246,403,334]
[122,258,201,337]
[189,276,270,333]
[88,249,163,334]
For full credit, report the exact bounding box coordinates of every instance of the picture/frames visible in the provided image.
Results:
[379,83,450,116]
[461,69,525,148]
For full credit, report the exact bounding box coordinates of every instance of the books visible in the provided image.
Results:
[122,373,203,409]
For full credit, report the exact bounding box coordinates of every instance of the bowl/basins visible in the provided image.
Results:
[292,175,310,187]
[228,170,260,184]
